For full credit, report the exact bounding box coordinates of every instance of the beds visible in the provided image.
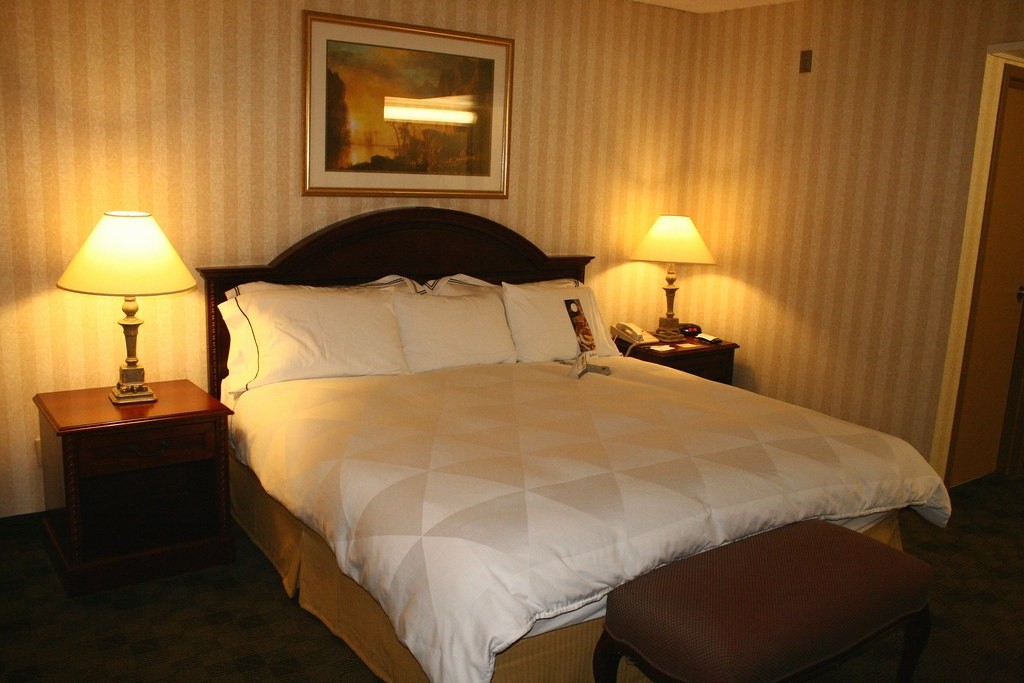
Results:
[192,206,953,683]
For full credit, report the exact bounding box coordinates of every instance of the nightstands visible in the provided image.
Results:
[31,379,238,597]
[611,333,740,385]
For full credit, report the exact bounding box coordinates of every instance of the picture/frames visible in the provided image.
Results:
[299,8,515,201]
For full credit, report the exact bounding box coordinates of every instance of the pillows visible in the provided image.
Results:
[224,273,427,301]
[499,281,622,364]
[392,294,520,374]
[421,272,584,296]
[215,289,411,395]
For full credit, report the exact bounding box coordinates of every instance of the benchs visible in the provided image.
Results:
[592,518,936,679]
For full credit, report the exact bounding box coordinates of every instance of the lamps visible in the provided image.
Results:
[54,209,197,406]
[629,214,716,345]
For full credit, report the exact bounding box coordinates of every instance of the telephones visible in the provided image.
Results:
[610,322,659,346]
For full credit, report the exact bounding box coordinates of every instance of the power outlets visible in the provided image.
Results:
[34,438,43,467]
[799,50,812,72]
